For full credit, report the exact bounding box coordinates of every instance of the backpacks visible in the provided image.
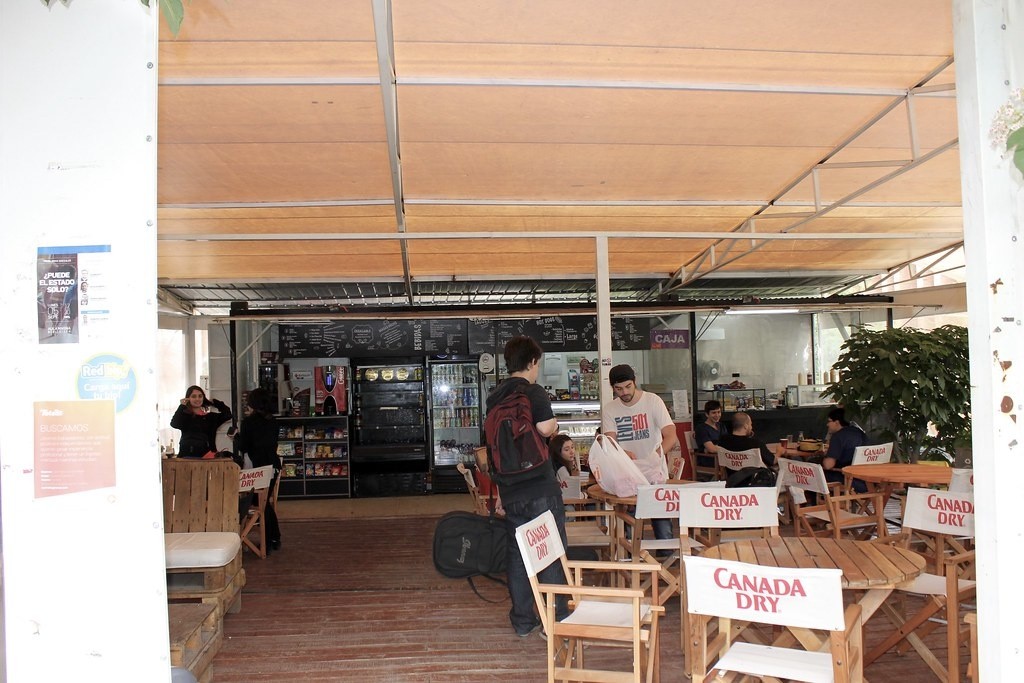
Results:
[483,381,554,488]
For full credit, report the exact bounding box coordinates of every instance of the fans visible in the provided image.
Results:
[701,359,721,391]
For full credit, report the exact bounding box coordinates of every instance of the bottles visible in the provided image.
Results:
[807,371,812,385]
[824,370,828,384]
[170,439,174,453]
[830,369,836,383]
[797,372,803,386]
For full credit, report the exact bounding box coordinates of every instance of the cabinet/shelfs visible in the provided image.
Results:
[274,415,351,499]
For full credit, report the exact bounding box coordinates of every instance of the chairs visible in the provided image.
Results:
[458,438,978,683]
[239,464,274,559]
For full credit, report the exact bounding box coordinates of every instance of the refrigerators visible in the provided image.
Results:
[426,354,483,493]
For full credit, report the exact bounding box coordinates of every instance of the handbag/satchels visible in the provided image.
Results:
[632,441,670,487]
[587,434,651,498]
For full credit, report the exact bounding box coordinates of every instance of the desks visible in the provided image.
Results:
[702,537,927,681]
[587,479,700,555]
[764,441,831,466]
[168,602,222,682]
[841,462,952,539]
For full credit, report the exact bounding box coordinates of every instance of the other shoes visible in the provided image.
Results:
[250,538,282,556]
[538,630,579,652]
[519,617,542,638]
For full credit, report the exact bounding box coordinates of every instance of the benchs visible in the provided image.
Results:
[161,458,247,617]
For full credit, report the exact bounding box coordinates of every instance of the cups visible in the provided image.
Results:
[780,438,788,449]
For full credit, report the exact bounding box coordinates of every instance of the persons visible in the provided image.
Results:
[481,334,572,642]
[694,399,729,466]
[232,389,283,551]
[168,385,232,456]
[717,412,786,465]
[601,364,679,583]
[547,424,601,521]
[807,405,877,506]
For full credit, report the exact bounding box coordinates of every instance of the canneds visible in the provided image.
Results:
[433,365,479,427]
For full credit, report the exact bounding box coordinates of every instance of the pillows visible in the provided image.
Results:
[473,470,501,507]
